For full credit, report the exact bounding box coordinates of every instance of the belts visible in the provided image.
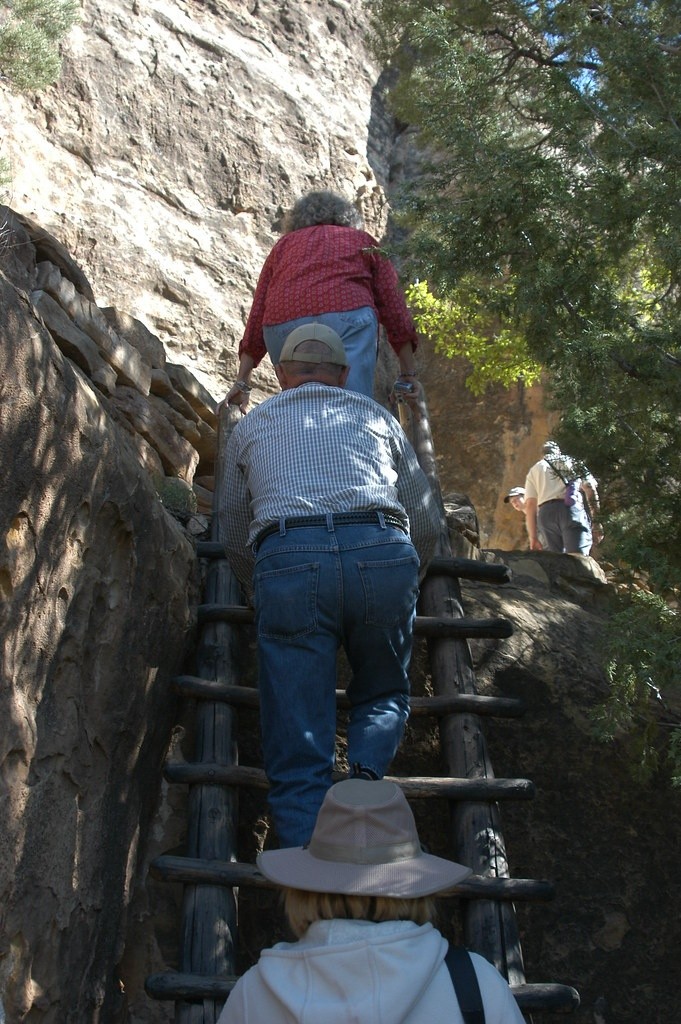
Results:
[255,512,409,552]
[540,499,566,508]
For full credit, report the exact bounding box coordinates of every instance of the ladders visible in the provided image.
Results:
[145,378,579,1023]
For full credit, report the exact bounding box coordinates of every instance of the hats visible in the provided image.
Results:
[256,777,472,900]
[504,487,525,504]
[279,322,347,368]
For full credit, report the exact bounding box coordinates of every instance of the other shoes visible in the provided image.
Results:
[349,763,377,780]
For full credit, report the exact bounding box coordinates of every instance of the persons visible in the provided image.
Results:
[220,321,442,848]
[216,779,527,1024]
[503,441,600,556]
[215,191,421,416]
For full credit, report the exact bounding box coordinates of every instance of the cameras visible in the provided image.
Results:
[393,381,414,404]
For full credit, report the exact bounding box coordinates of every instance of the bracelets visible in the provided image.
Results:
[235,380,252,394]
[398,369,419,378]
